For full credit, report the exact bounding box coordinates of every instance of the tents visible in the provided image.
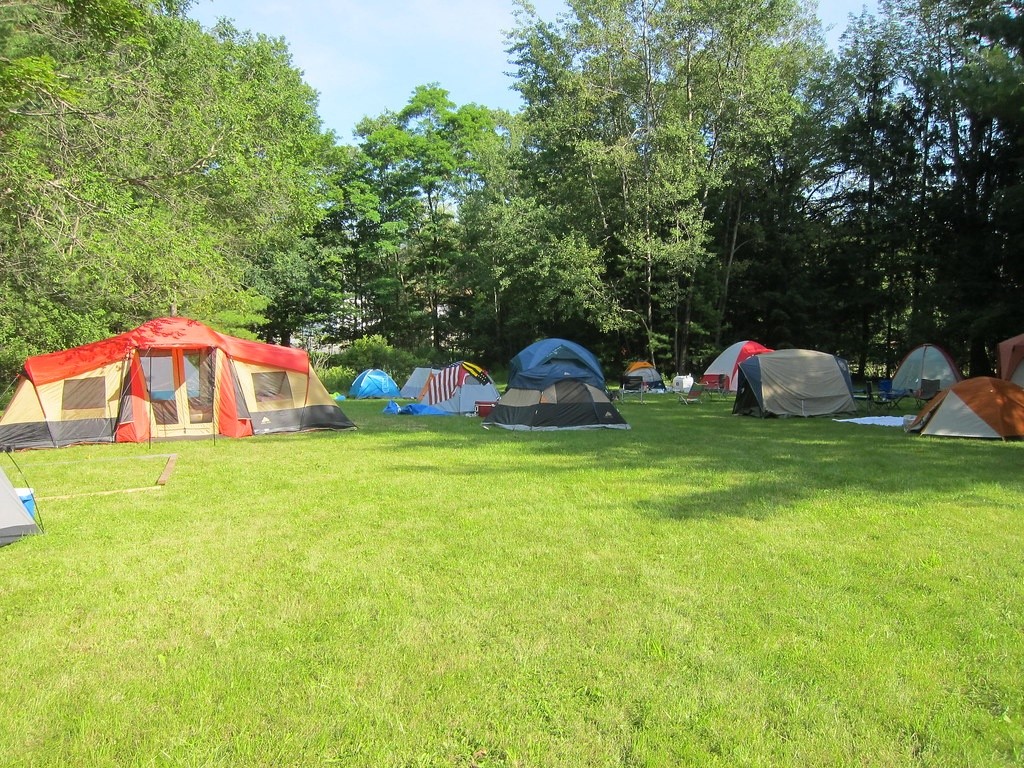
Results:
[996,333,1024,387]
[731,349,858,418]
[0,465,41,548]
[698,341,774,392]
[400,366,442,399]
[907,375,1024,439]
[348,368,402,398]
[420,361,500,413]
[483,364,631,430]
[507,338,606,387]
[0,318,356,449]
[890,342,965,392]
[625,362,665,392]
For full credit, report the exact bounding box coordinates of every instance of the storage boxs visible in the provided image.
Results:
[15,488,35,518]
[475,401,496,417]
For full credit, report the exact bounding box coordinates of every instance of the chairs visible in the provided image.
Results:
[708,373,727,401]
[678,381,709,405]
[915,378,941,409]
[622,376,643,404]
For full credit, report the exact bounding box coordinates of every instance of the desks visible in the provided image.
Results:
[873,393,910,410]
